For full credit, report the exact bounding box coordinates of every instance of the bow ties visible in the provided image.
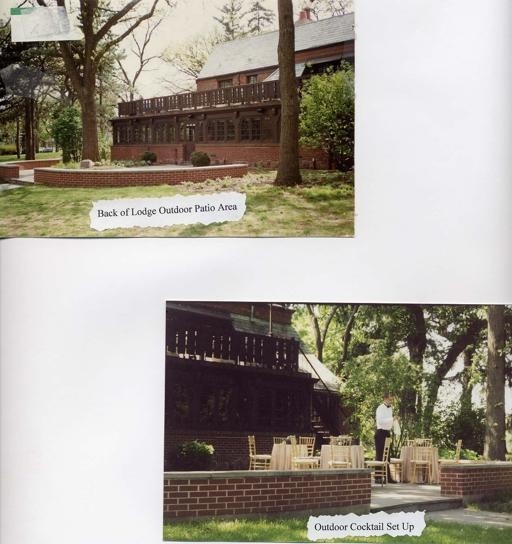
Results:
[386,405,391,408]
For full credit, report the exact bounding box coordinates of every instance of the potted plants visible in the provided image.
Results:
[176,440,214,469]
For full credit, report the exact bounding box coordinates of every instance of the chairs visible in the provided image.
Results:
[247,435,463,486]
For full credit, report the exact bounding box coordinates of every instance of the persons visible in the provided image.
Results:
[374,393,397,483]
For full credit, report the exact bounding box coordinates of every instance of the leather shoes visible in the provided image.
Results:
[375,477,397,484]
